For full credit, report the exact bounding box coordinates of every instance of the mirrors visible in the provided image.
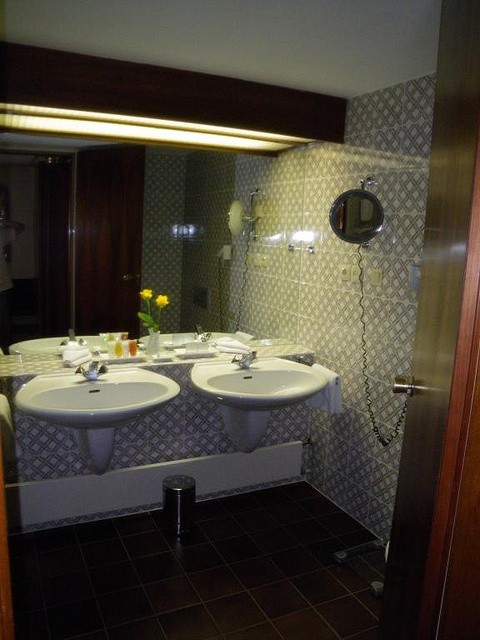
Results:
[327,177,386,243]
[1,130,304,364]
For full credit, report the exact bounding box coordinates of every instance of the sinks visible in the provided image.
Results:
[139,332,253,347]
[205,367,324,396]
[14,336,117,352]
[26,380,171,411]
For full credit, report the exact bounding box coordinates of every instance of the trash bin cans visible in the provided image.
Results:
[164,476,196,535]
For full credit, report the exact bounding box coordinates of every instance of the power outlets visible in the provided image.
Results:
[408,266,421,292]
[217,242,233,263]
[349,265,364,283]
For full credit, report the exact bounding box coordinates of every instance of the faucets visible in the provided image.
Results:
[59,328,88,346]
[74,359,110,383]
[192,323,212,342]
[230,350,260,371]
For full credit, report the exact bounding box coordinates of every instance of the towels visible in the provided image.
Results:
[211,337,235,347]
[217,340,251,355]
[62,347,92,368]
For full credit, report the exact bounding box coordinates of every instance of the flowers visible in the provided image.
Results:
[149,287,169,331]
[135,288,154,330]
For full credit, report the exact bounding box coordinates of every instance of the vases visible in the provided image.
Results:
[147,329,161,357]
